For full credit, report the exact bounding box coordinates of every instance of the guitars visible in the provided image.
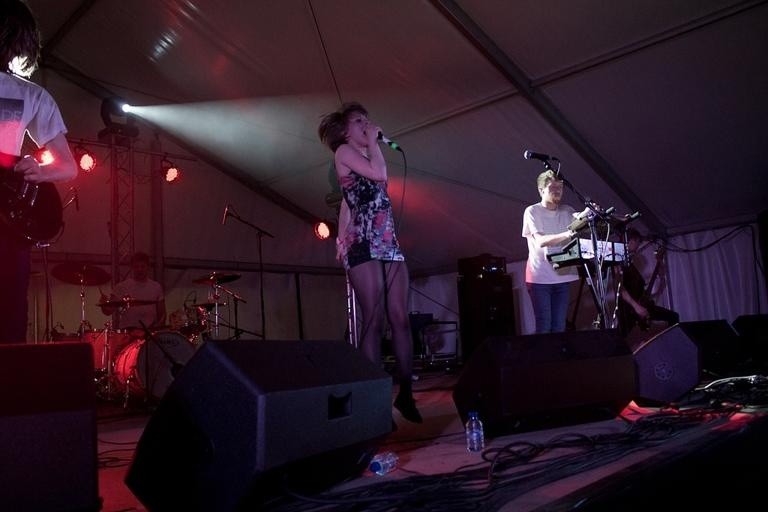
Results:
[0,149,63,247]
[632,245,665,330]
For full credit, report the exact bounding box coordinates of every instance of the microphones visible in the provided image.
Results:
[523,149,559,161]
[74,194,80,211]
[635,240,652,254]
[376,130,403,153]
[222,205,229,226]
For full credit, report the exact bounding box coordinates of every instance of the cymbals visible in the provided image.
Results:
[50,262,111,286]
[96,300,156,306]
[191,303,225,307]
[192,272,241,285]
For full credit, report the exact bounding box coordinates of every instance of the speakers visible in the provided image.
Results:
[0,341,100,512]
[451,329,634,439]
[458,276,514,344]
[632,318,742,402]
[730,314,767,377]
[123,339,392,512]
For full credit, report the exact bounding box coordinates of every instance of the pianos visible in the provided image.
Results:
[546,235,631,272]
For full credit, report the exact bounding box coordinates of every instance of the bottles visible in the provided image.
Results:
[466,411,483,452]
[370,453,400,476]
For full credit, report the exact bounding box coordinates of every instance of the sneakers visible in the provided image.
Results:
[394,394,422,424]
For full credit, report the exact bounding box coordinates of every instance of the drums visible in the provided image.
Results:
[114,332,197,402]
[131,327,148,338]
[83,331,130,372]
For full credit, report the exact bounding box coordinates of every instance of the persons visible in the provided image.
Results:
[520,169,602,335]
[0,0,79,344]
[317,100,423,432]
[98,250,166,331]
[610,228,678,337]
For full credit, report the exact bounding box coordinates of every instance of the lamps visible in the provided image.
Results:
[70,143,96,171]
[30,142,52,160]
[162,157,180,182]
[313,221,329,239]
